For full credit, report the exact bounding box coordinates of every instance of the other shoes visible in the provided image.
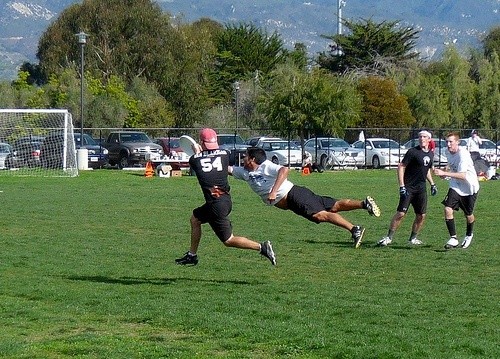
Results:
[444,237,459,249]
[408,238,422,244]
[462,236,472,248]
[380,237,392,246]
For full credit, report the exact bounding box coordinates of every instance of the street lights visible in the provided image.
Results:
[74,32,91,148]
[232,82,241,167]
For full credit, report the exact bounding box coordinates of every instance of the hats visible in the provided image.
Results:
[200,128,219,150]
[419,131,431,139]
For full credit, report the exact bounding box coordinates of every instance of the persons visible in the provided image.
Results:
[466,133,482,162]
[174,128,277,268]
[228,146,381,249]
[378,131,437,246]
[302,155,323,174]
[434,132,481,249]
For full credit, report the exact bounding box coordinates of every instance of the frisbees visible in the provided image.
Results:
[179,135,200,156]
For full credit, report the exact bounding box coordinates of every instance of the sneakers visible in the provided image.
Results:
[352,226,365,249]
[260,240,276,266]
[364,196,380,217]
[175,251,198,266]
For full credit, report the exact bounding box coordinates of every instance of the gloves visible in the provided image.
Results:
[400,186,409,199]
[431,184,438,196]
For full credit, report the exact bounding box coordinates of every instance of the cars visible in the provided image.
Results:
[255,138,312,166]
[39,131,109,169]
[105,131,164,170]
[0,136,45,169]
[350,138,409,169]
[403,139,447,166]
[304,138,364,171]
[153,137,191,170]
[217,133,252,167]
[458,138,500,162]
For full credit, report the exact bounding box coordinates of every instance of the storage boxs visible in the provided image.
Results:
[153,165,182,178]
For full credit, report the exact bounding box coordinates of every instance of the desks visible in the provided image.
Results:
[148,156,192,176]
